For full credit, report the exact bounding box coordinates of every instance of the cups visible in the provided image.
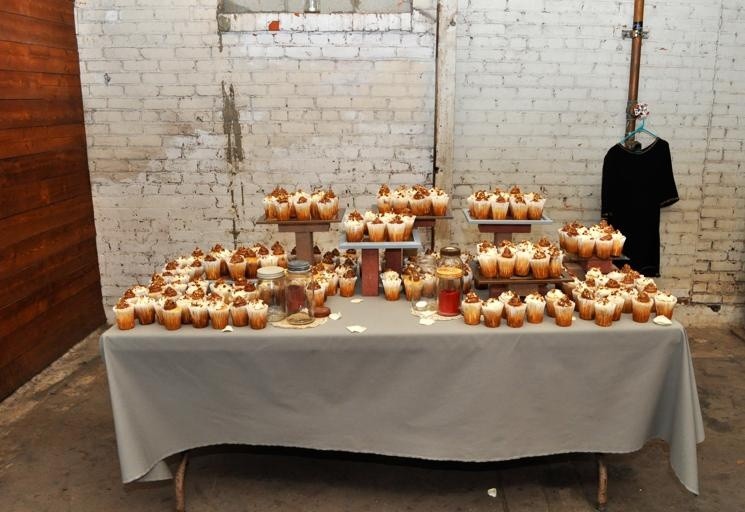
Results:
[261,185,452,308]
[112,243,267,331]
[461,187,677,327]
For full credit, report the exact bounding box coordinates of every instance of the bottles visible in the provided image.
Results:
[436,247,461,318]
[257,259,315,325]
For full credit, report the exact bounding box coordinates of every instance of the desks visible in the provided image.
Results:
[99,261,705,512]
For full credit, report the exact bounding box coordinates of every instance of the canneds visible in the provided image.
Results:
[256,265,290,322]
[285,260,314,325]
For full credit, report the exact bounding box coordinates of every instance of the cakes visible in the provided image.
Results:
[152,240,286,283]
[308,246,360,308]
[466,184,546,221]
[381,248,472,301]
[112,284,268,331]
[469,239,567,275]
[455,284,576,328]
[554,220,628,258]
[344,207,415,242]
[260,187,338,221]
[373,187,447,216]
[563,262,676,326]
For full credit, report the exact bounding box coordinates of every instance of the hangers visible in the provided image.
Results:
[616,117,658,145]
